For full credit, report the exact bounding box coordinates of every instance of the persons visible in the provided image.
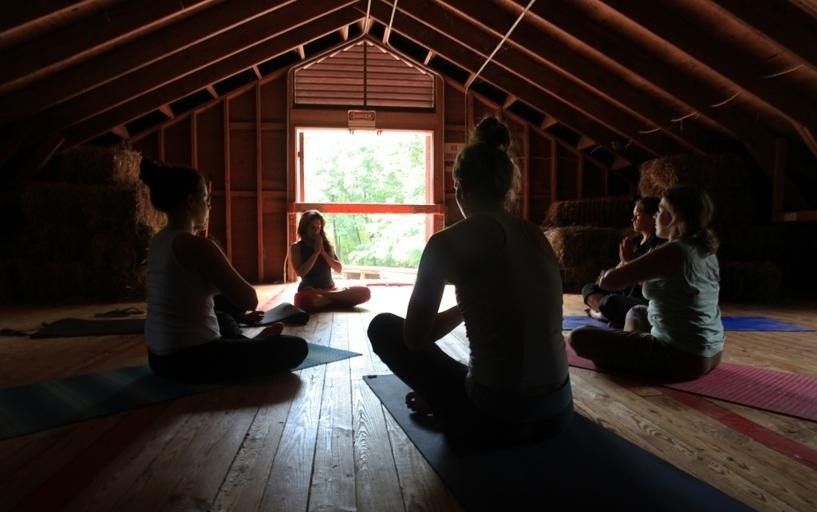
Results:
[564,183,729,386]
[362,110,578,448]
[288,210,370,316]
[133,156,308,385]
[577,194,662,330]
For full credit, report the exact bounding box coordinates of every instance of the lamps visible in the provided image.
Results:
[346,109,376,129]
